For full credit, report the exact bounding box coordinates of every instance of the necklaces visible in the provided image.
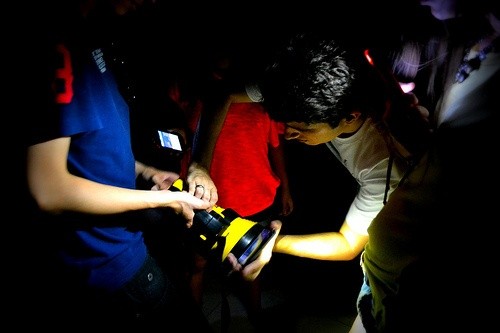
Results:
[456,34,500,81]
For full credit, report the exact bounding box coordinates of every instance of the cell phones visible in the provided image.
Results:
[157,130,187,154]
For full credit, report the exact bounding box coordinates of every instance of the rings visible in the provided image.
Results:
[196,184,205,188]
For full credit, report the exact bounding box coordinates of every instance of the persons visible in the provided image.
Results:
[180,39,293,333]
[348,0,500,333]
[186,35,425,333]
[27,0,207,333]
[151,84,207,307]
